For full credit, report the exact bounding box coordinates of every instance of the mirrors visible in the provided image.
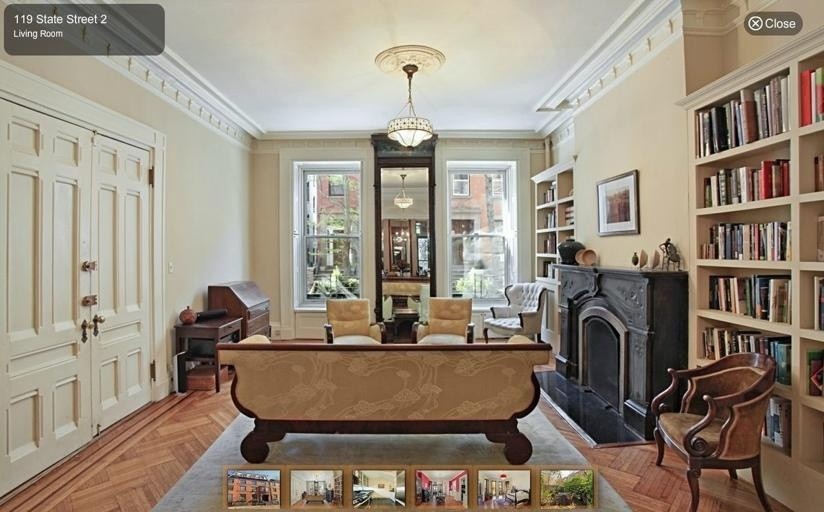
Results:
[368,133,440,322]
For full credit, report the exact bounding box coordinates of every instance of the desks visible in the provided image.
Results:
[171,308,244,398]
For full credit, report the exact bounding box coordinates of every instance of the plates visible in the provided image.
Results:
[651,250,660,271]
[638,250,649,269]
[582,250,597,266]
[575,250,588,265]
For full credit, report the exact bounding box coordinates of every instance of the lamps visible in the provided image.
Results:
[392,173,414,209]
[373,43,449,150]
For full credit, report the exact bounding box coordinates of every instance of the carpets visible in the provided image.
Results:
[146,407,638,512]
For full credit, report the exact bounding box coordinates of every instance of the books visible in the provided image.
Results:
[542,180,574,279]
[693,67,824,458]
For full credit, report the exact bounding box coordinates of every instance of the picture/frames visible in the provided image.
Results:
[593,167,642,238]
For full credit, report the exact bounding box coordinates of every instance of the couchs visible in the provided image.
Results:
[207,334,555,466]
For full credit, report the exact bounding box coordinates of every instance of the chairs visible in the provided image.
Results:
[647,344,782,512]
[323,296,386,347]
[410,295,476,345]
[481,281,548,346]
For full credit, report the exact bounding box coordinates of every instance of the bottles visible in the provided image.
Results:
[415,266,426,278]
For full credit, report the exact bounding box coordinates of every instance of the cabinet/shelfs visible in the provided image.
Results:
[529,158,575,289]
[208,281,271,338]
[669,23,824,476]
